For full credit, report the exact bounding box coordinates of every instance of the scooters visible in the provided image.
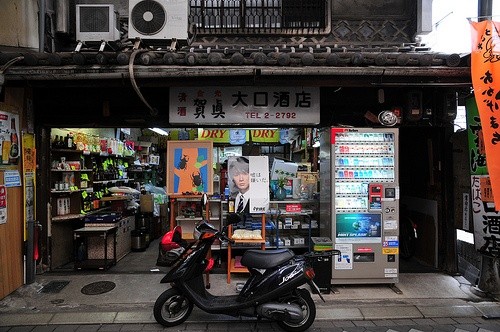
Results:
[153,194,341,332]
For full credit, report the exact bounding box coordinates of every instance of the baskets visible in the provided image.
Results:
[156,242,186,266]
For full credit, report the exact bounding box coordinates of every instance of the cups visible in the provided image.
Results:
[2,141,11,164]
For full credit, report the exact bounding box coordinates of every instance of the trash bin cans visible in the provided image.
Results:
[131,229,146,252]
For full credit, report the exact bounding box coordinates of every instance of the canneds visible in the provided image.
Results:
[54,181,70,191]
[335,134,394,178]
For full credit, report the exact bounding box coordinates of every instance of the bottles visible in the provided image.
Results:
[9,117,20,164]
[54,173,75,191]
[57,194,70,216]
[50,133,76,150]
[77,131,148,211]
[335,133,395,212]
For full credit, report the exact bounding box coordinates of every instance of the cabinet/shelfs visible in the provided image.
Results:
[50,148,134,220]
[170,165,265,284]
[266,200,319,250]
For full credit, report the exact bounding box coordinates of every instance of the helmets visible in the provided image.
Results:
[161,226,182,251]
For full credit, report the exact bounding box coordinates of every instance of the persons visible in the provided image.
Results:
[229,157,249,212]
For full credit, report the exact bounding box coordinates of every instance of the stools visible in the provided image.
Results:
[73,227,118,272]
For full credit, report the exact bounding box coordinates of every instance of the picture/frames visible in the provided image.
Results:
[167,140,213,197]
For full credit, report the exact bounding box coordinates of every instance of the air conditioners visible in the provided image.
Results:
[129,0,190,39]
[76,4,121,41]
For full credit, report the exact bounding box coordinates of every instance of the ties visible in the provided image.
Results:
[236,195,243,213]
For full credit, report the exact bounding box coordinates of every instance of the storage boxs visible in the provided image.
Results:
[279,218,318,246]
